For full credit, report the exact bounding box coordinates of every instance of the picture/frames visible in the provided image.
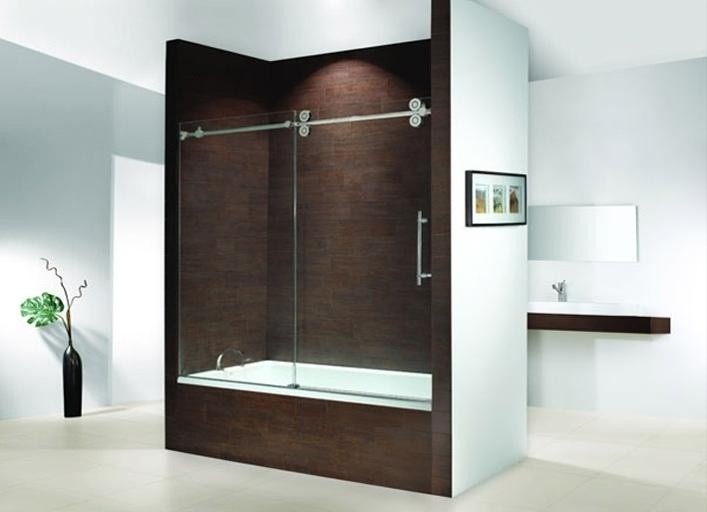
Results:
[466,168,527,227]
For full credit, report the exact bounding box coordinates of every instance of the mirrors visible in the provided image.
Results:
[527,203,639,265]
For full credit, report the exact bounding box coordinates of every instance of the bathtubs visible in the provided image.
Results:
[176,361,432,489]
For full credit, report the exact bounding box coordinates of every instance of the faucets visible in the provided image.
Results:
[552,280,567,302]
[217,348,244,371]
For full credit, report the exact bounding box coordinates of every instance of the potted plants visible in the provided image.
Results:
[18,257,89,417]
